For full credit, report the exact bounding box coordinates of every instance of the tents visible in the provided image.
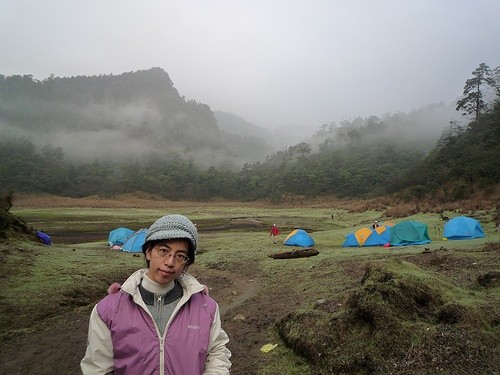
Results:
[108,227,135,247]
[342,220,432,246]
[283,229,314,248]
[444,215,485,240]
[36,231,51,245]
[121,227,148,253]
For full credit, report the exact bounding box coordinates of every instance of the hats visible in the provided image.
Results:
[145,214,198,264]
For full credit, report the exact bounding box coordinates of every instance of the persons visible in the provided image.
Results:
[270,224,280,244]
[80,215,232,375]
[372,221,379,230]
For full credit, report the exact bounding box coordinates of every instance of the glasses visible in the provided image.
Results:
[151,243,191,265]
